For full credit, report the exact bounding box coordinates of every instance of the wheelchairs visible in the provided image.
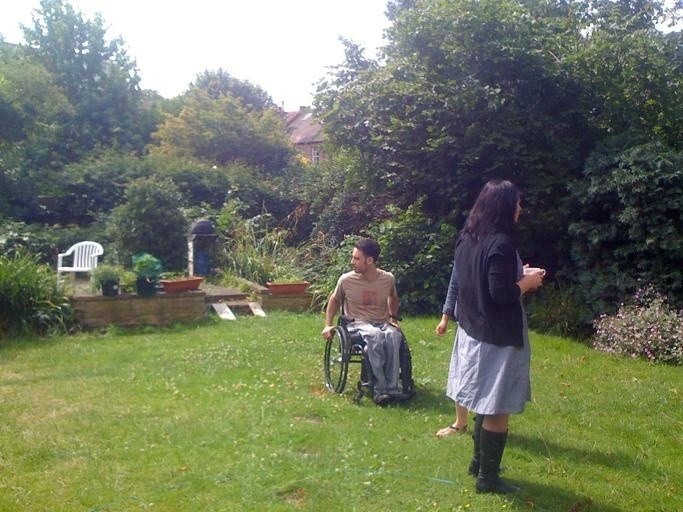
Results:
[322,285,416,407]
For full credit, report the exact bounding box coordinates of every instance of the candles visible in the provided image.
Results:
[87,253,204,297]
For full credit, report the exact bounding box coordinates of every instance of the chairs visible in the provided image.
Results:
[55,239,104,296]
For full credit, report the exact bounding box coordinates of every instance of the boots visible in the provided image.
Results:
[469,413,523,495]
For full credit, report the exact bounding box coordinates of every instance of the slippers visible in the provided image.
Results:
[434,422,468,438]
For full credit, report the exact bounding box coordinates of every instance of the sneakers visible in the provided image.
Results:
[374,392,410,405]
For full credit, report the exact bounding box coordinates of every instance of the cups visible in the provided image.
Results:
[523,268,547,276]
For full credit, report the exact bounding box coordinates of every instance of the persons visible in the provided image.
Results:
[443,178,547,492]
[321,238,411,404]
[435,257,468,437]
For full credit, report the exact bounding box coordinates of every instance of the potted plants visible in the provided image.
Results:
[265,275,310,295]
